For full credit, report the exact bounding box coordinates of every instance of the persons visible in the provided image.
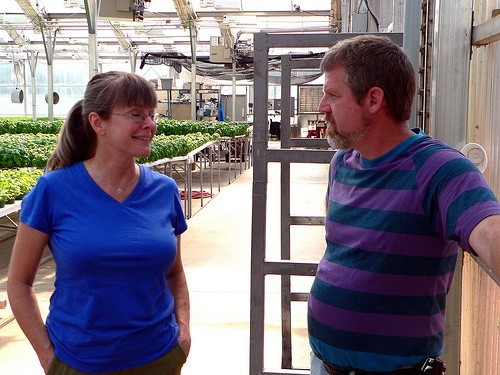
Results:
[307,36,500,375]
[5,71,191,375]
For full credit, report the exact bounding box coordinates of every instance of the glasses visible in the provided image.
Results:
[98,110,157,125]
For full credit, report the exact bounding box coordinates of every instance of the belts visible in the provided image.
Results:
[324,363,417,375]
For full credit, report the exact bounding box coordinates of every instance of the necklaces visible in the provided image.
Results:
[90,159,139,195]
